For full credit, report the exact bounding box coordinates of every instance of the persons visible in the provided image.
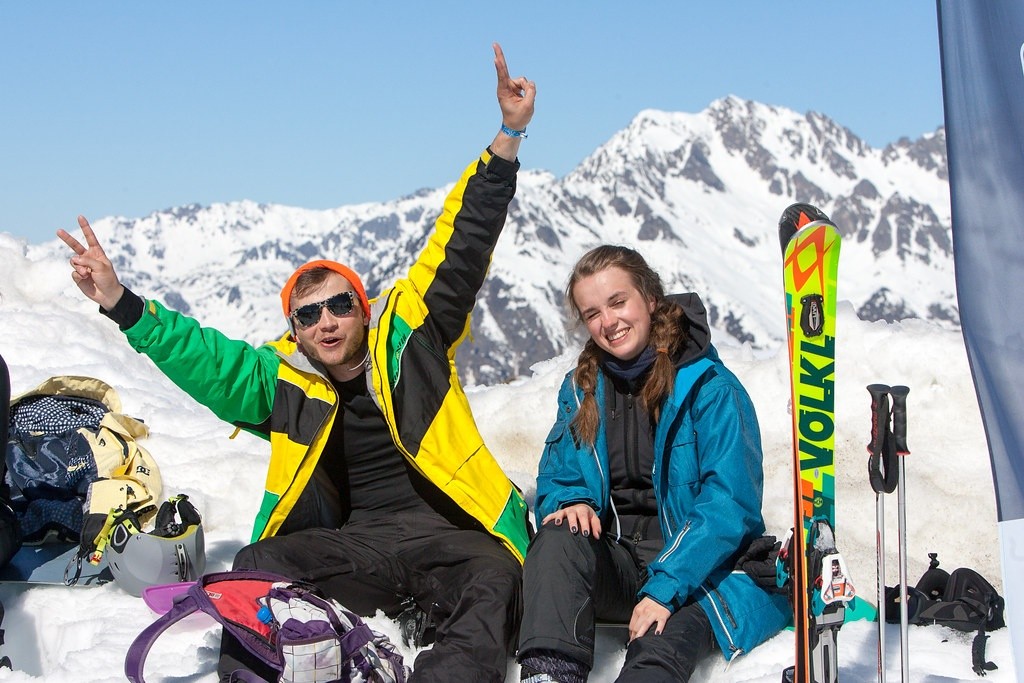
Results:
[57,42,536,683]
[517,245,794,683]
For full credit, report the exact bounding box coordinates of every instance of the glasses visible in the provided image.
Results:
[287,290,359,328]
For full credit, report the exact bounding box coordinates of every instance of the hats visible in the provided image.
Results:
[279,260,371,341]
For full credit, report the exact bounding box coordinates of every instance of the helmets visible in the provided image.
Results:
[106,493,205,596]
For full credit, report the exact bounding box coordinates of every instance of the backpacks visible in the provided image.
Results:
[125,569,411,683]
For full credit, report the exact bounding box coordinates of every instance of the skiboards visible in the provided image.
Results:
[773,203,860,683]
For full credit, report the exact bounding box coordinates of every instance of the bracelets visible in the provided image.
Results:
[501,123,528,139]
